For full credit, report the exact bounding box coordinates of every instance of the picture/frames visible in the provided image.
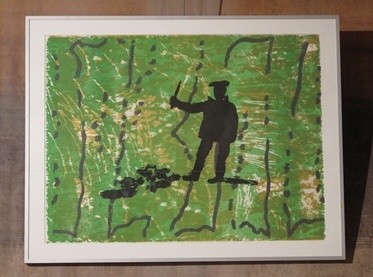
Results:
[23,15,347,265]
